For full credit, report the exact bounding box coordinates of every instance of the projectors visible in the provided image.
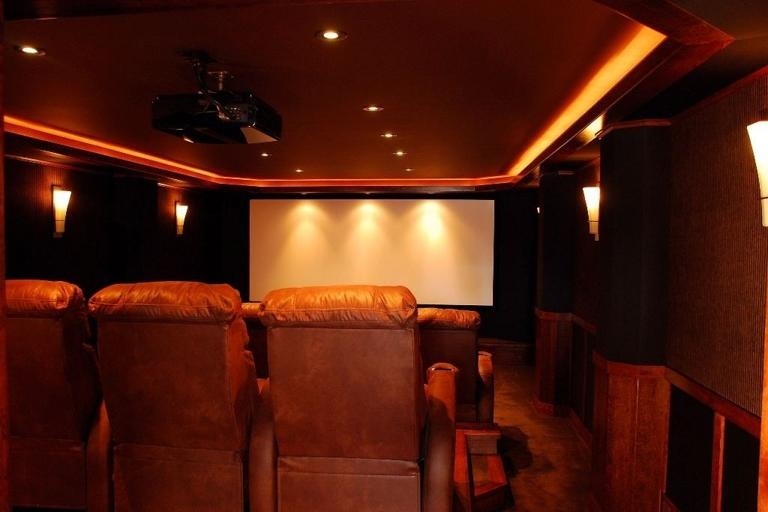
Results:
[151,93,282,144]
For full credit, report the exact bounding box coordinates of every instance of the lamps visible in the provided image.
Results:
[176,202,189,235]
[748,121,768,228]
[52,187,72,238]
[583,186,600,234]
